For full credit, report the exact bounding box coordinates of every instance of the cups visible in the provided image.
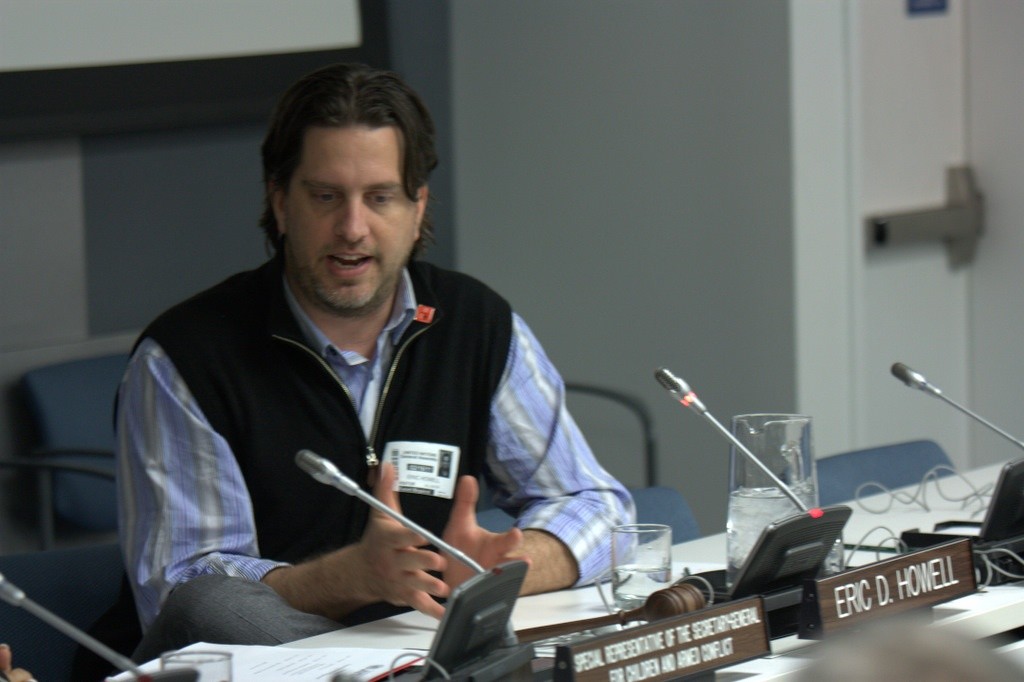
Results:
[725,413,821,590]
[161,650,233,682]
[610,523,674,610]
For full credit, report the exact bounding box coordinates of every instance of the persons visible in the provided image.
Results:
[109,59,640,650]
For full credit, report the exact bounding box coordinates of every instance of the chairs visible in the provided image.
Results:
[566,378,699,556]
[13,352,163,546]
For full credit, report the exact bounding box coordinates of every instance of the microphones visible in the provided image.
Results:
[293,448,486,574]
[653,365,805,510]
[890,361,1023,451]
[0,572,144,679]
